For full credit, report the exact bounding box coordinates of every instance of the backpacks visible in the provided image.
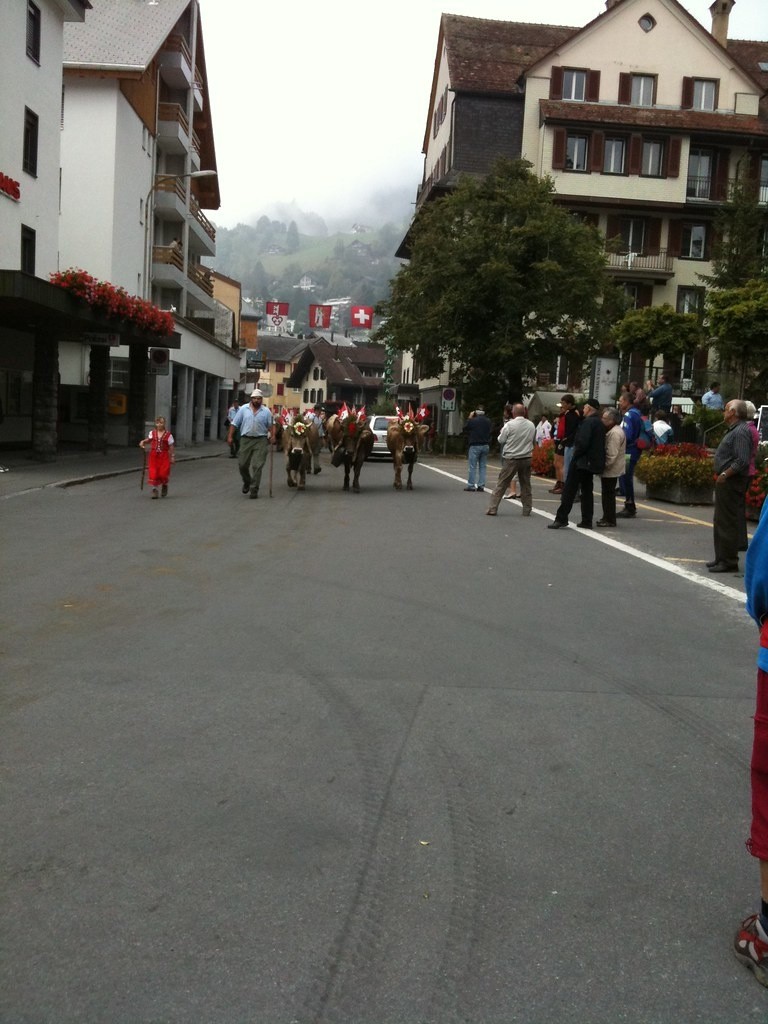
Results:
[628,409,657,450]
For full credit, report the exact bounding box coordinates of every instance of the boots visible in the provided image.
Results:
[616,501,637,518]
[549,481,565,493]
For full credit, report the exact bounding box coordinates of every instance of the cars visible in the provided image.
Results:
[366,414,398,454]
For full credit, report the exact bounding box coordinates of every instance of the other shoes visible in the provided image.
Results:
[161,485,167,495]
[250,494,257,498]
[152,489,159,499]
[706,559,738,573]
[596,518,616,527]
[464,487,475,491]
[577,521,593,529]
[477,486,484,491]
[547,521,569,529]
[242,479,251,493]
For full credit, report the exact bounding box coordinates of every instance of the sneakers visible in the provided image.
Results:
[734,914,768,989]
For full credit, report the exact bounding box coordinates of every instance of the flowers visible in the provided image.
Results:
[531,438,558,474]
[635,440,716,489]
[49,268,175,343]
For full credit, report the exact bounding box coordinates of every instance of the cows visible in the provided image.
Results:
[335,418,374,493]
[284,419,313,491]
[291,414,322,474]
[326,414,340,453]
[387,419,429,490]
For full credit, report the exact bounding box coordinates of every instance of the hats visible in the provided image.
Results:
[587,398,599,411]
[250,389,264,398]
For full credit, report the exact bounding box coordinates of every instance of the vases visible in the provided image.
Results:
[642,479,714,504]
[542,465,556,477]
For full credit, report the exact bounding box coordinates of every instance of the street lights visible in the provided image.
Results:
[142,170,219,299]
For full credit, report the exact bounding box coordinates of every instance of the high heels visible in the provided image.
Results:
[504,493,516,499]
[515,496,521,498]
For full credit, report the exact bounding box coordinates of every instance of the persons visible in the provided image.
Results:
[706,400,759,572]
[427,422,437,454]
[303,404,325,474]
[139,417,176,498]
[228,390,277,498]
[548,375,695,528]
[227,399,242,458]
[486,405,535,517]
[732,493,768,988]
[502,404,522,499]
[534,415,552,447]
[701,382,726,411]
[464,404,493,491]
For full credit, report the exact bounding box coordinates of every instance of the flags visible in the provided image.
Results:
[266,301,373,328]
[393,402,430,423]
[337,401,367,425]
[277,404,313,428]
